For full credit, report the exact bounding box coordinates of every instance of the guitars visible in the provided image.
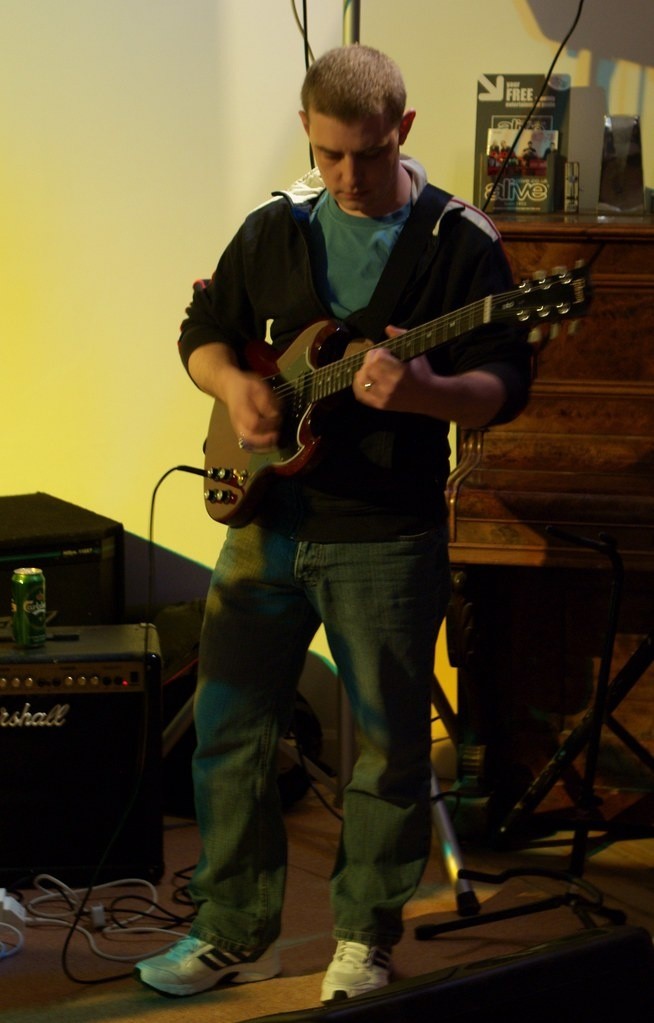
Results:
[202,257,596,528]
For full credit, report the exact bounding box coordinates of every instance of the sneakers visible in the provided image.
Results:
[134,936,281,998]
[320,939,392,1003]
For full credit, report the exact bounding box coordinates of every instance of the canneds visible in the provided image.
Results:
[10,567,48,649]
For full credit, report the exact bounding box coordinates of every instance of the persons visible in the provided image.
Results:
[130,44,536,1005]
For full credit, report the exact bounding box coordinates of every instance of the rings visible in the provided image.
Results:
[364,380,376,391]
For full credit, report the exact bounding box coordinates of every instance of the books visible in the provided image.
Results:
[474,73,571,214]
